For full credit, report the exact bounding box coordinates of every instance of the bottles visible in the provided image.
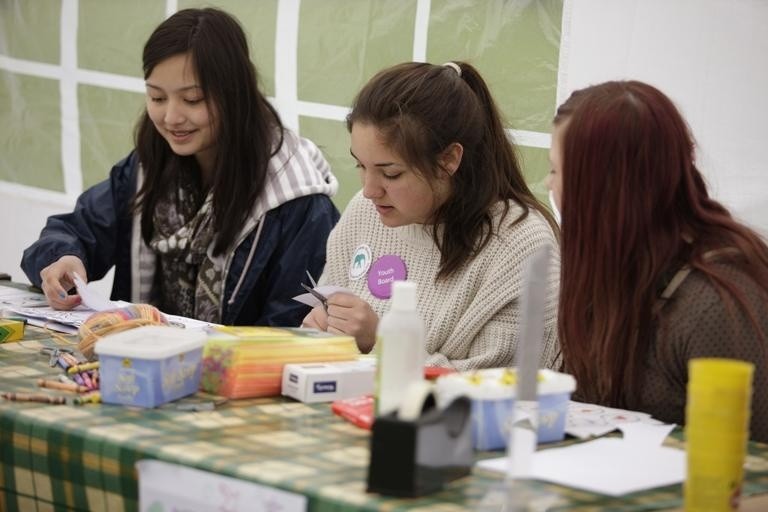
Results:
[373,281,427,421]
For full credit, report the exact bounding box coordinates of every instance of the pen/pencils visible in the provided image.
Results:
[1,352,101,405]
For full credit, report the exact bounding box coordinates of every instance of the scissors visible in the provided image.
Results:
[40,346,74,366]
[300,269,328,313]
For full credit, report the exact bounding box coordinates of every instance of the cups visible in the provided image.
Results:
[682,358,755,512]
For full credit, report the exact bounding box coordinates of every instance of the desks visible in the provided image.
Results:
[0,280,768,511]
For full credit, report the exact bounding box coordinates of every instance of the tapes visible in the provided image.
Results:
[397,378,441,421]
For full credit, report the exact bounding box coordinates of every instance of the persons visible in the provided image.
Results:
[544,80,768,443]
[299,61,564,373]
[21,8,342,328]
